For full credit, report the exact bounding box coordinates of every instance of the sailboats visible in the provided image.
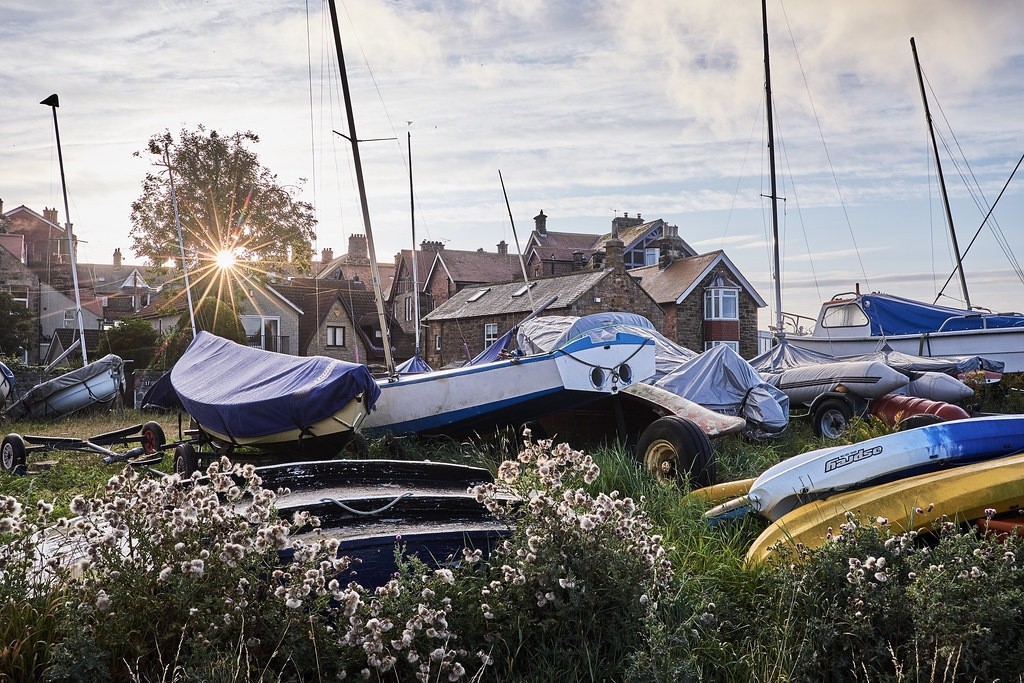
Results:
[0,0,1023,595]
